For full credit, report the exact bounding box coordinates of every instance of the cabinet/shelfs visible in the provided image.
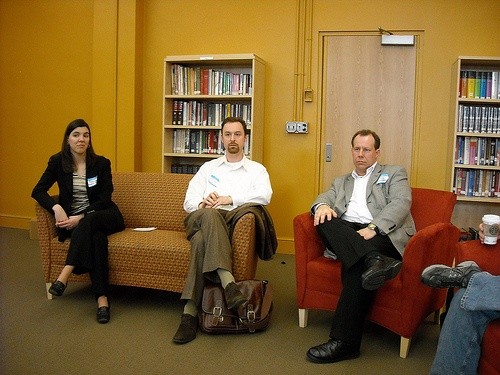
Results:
[450,56,500,203]
[161,53,265,173]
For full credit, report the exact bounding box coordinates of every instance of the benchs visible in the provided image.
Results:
[36,172,258,298]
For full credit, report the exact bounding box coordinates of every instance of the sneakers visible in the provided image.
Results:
[420,260,481,289]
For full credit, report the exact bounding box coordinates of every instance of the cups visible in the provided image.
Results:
[481,213,500,245]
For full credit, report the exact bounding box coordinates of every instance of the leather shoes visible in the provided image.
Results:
[360,252,402,290]
[306,338,360,365]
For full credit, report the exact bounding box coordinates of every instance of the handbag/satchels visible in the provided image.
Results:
[199,280,274,334]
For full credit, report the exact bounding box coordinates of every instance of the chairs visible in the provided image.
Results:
[294,187,462,359]
[454,239,500,375]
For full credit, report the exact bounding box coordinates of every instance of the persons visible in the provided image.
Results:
[421,223,500,375]
[171,117,273,344]
[31,118,125,324]
[307,129,417,363]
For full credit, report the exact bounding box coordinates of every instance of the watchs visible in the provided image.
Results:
[368,224,379,236]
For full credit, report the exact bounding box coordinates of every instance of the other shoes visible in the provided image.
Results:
[96,302,110,324]
[173,314,197,345]
[48,278,68,296]
[224,281,248,310]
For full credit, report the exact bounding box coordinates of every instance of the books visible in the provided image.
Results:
[170,64,252,174]
[452,70,500,199]
